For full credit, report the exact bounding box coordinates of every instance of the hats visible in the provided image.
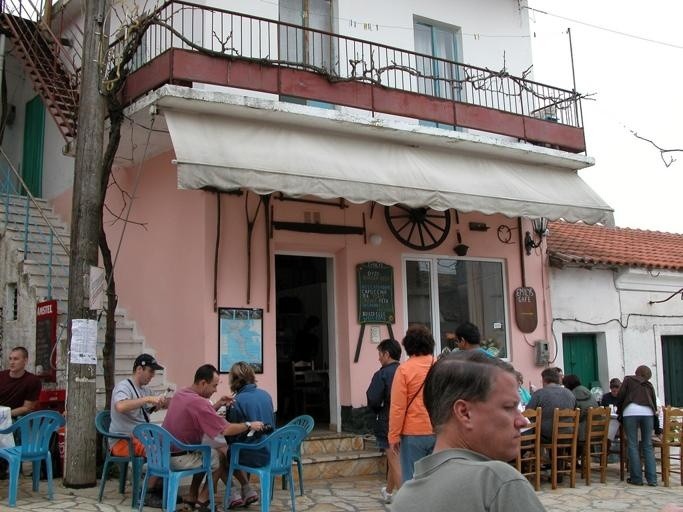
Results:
[134,354,163,370]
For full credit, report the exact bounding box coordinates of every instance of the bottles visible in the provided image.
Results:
[145,388,172,413]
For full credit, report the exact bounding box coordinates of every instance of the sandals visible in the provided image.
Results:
[184,501,195,512]
[195,500,221,512]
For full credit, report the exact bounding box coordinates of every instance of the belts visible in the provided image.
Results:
[171,450,192,456]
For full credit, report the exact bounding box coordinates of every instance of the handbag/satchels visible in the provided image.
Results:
[654,415,660,434]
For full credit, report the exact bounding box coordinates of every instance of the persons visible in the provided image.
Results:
[1,347,40,479]
[107,353,171,508]
[366,323,493,503]
[390,348,546,511]
[160,364,264,511]
[514,366,658,486]
[219,362,274,510]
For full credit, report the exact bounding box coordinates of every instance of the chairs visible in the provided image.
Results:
[540,405,582,490]
[95,409,145,510]
[577,406,613,486]
[133,423,215,512]
[652,404,683,490]
[225,424,308,512]
[0,409,67,508]
[271,415,314,501]
[511,406,543,491]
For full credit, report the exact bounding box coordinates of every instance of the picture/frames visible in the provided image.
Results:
[217,305,264,374]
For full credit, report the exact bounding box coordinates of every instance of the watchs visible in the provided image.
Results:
[245,421,252,429]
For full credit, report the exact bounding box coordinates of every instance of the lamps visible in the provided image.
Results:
[523,217,549,255]
[368,232,383,248]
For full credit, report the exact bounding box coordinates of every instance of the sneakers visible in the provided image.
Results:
[627,478,643,484]
[381,487,393,503]
[144,491,162,508]
[229,500,244,508]
[244,495,258,504]
